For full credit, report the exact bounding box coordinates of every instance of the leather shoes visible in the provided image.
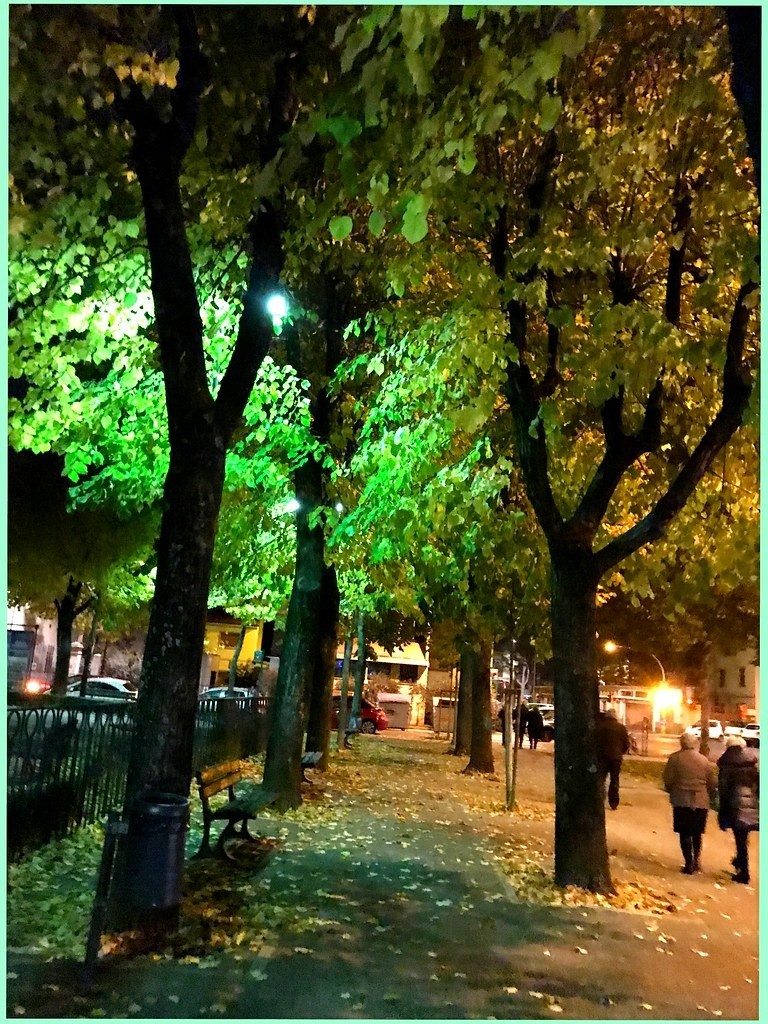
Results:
[681,864,699,875]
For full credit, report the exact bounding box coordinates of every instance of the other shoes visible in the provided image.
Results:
[519,744,522,749]
[530,745,532,749]
[732,858,749,884]
[534,744,536,749]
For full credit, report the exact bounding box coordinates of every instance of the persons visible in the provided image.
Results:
[664,733,717,875]
[499,699,543,751]
[599,709,630,810]
[716,737,759,884]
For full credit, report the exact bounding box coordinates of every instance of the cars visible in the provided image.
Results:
[684,718,760,742]
[331,695,389,734]
[199,687,266,714]
[37,674,138,701]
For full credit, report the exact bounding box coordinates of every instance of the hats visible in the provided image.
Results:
[726,737,746,748]
[681,733,699,749]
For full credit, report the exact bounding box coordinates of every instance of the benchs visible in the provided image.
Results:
[300,751,324,794]
[189,757,281,861]
[344,729,358,749]
